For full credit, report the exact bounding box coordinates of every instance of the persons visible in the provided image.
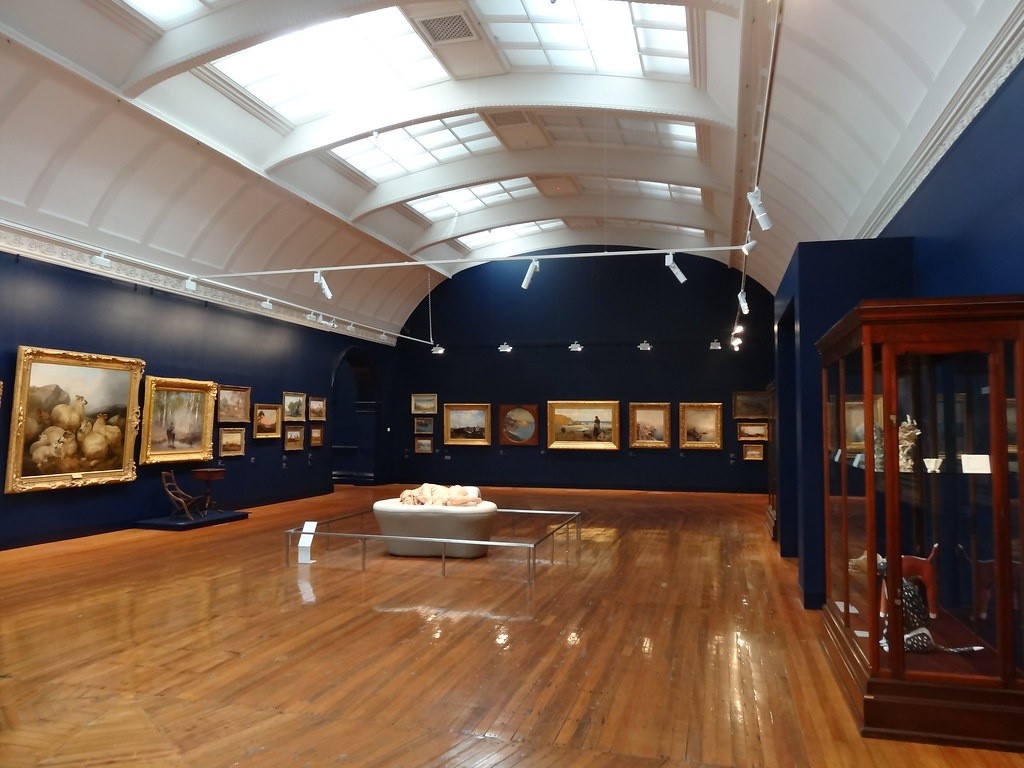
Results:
[400,483,482,506]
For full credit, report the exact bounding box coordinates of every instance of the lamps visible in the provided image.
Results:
[741,241,756,256]
[521,259,538,289]
[94,255,445,355]
[568,341,584,351]
[665,254,688,284]
[738,293,749,314]
[746,187,774,232]
[498,342,513,352]
[710,340,721,349]
[638,341,653,350]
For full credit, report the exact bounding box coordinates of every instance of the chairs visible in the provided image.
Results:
[161,470,204,520]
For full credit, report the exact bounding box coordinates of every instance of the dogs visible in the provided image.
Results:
[848,550,986,654]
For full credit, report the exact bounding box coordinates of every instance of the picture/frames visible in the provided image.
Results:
[310,425,323,447]
[411,394,437,415]
[842,392,1020,454]
[628,402,671,449]
[138,376,218,465]
[282,392,307,422]
[6,346,144,495]
[498,404,539,445]
[443,404,491,446]
[219,428,245,457]
[414,417,433,435]
[284,426,304,451]
[547,401,621,451]
[414,436,433,453]
[253,404,283,439]
[677,402,722,449]
[308,397,327,422]
[215,384,252,424]
[732,391,775,460]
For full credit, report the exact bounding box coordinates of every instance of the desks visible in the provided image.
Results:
[373,498,497,558]
[190,468,227,516]
